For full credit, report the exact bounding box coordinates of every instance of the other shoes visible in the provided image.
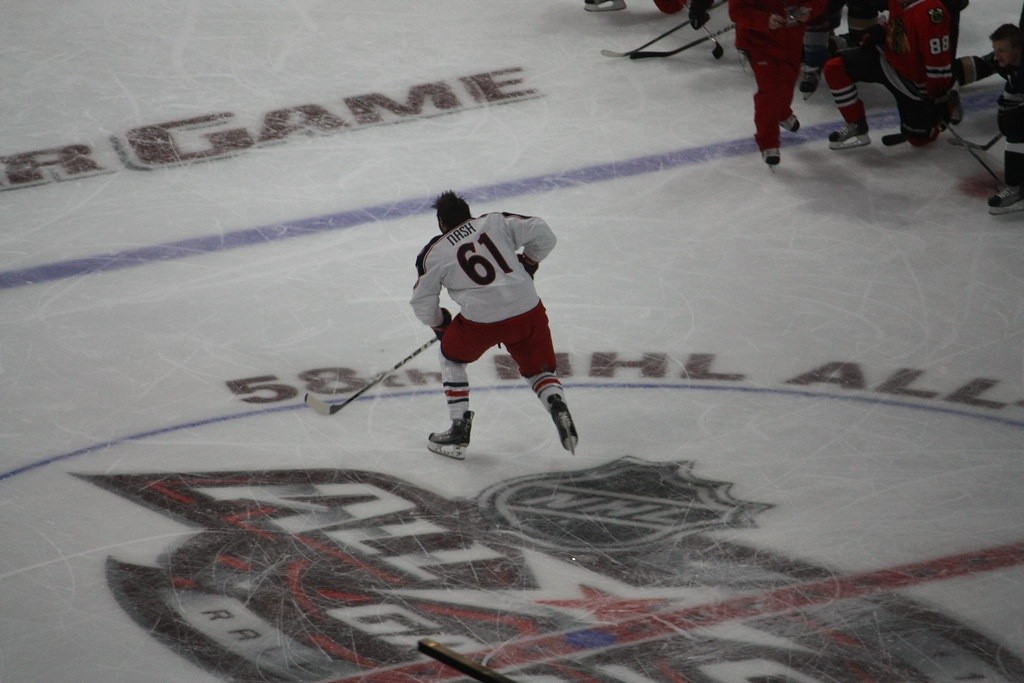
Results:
[584,0,627,11]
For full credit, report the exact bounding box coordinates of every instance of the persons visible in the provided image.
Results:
[410,189,578,460]
[582,0,1024,216]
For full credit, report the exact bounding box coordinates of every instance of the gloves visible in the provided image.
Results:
[432,307,453,341]
[861,23,886,49]
[930,92,946,117]
[688,4,706,30]
[516,253,539,279]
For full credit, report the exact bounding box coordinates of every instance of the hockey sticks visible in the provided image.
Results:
[303,336,442,415]
[946,129,1006,153]
[599,1,735,62]
[946,124,1004,188]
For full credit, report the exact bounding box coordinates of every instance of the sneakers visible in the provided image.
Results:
[779,113,800,134]
[426,411,475,459]
[986,184,1024,214]
[546,393,578,456]
[828,117,870,149]
[762,146,780,173]
[947,89,964,126]
[800,65,821,101]
